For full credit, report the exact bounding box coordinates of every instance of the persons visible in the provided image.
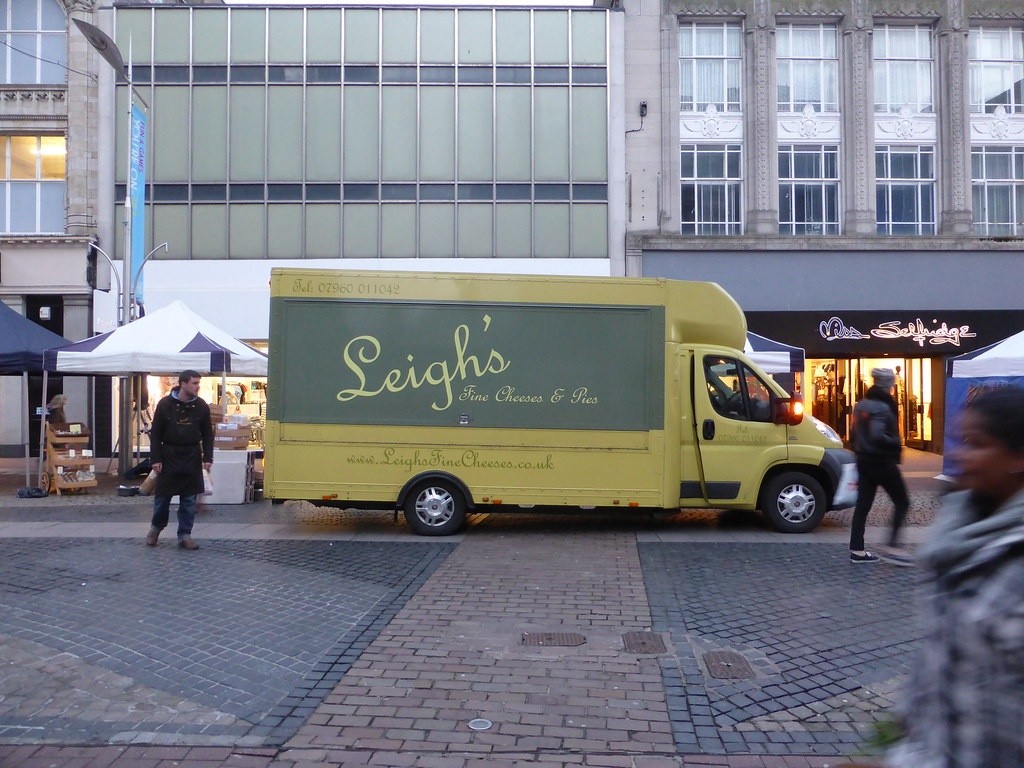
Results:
[888,384,1024,768]
[892,365,907,438]
[51,395,67,423]
[146,369,212,551]
[849,368,916,567]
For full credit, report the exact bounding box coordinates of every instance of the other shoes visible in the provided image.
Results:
[147,530,159,546]
[179,538,200,550]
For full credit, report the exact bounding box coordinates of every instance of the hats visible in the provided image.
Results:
[871,367,895,388]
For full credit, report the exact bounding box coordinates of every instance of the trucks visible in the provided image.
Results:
[261,264,857,536]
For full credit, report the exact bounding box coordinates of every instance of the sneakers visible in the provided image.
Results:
[881,549,916,567]
[850,551,880,563]
